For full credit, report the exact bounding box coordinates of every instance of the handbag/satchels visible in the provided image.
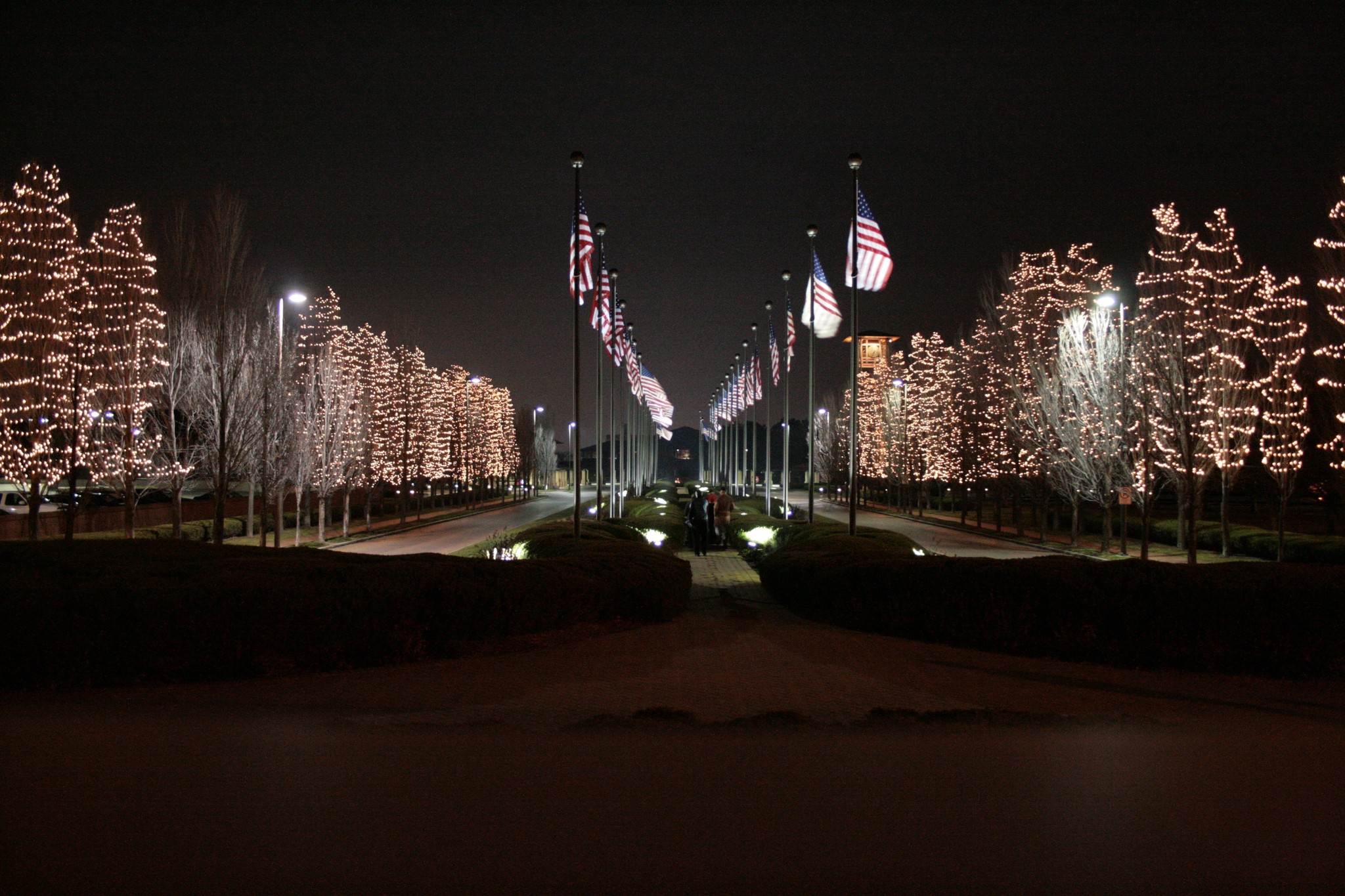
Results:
[717,541,721,545]
[685,520,695,532]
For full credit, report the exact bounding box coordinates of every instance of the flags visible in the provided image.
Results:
[844,185,893,291]
[768,319,780,386]
[588,242,612,337]
[569,184,595,305]
[783,294,795,372]
[801,246,843,338]
[701,343,763,443]
[605,290,674,442]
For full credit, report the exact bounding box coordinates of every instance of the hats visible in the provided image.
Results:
[719,487,728,491]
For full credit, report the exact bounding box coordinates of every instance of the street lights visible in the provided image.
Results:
[780,423,790,493]
[818,408,831,500]
[892,378,907,514]
[1094,294,1128,555]
[464,377,482,510]
[568,422,575,491]
[259,288,308,547]
[532,406,545,498]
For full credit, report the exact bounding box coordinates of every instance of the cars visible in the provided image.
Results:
[0,486,75,517]
[46,487,243,508]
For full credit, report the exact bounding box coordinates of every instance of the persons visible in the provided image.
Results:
[714,487,735,546]
[684,489,717,549]
[688,490,709,557]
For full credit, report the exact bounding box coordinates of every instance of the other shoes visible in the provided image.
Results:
[702,551,707,555]
[707,544,711,548]
[723,545,727,549]
[695,552,700,556]
